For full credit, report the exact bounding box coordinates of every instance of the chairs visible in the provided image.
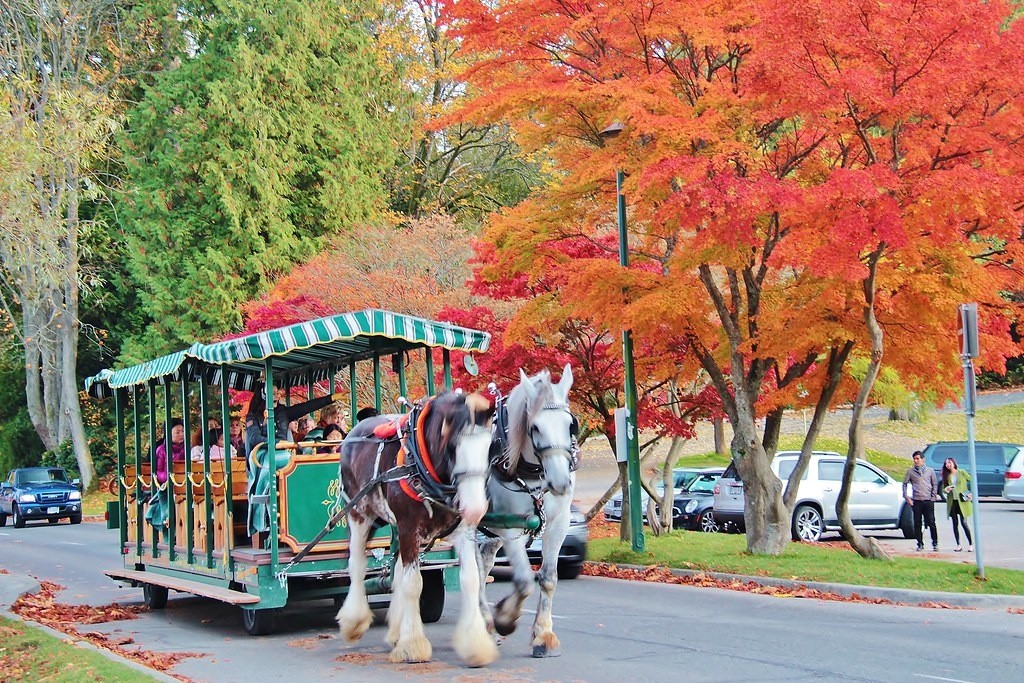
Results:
[675,474,686,488]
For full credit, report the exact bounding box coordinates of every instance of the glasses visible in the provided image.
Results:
[328,406,341,416]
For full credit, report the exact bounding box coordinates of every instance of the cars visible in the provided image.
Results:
[602,467,726,524]
[1001,446,1024,502]
[656,467,723,533]
[476,500,588,581]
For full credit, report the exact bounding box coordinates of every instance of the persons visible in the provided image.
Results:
[48,473,59,480]
[289,405,349,456]
[356,407,379,422]
[244,379,350,491]
[144,417,244,527]
[940,458,973,551]
[902,451,939,551]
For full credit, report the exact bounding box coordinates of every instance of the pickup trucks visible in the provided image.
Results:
[0,467,83,529]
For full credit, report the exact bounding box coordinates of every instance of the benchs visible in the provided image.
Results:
[123,457,250,536]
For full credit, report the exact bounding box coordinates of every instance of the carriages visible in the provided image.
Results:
[82,308,581,668]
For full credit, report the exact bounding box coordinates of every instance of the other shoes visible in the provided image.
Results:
[931,545,937,551]
[917,545,924,552]
[968,545,974,552]
[954,545,962,552]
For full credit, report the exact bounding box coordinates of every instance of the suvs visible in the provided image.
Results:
[923,442,1024,502]
[714,450,927,543]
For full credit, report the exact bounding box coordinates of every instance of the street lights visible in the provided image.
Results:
[597,121,645,555]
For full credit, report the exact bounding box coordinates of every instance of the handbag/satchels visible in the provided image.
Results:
[960,489,971,503]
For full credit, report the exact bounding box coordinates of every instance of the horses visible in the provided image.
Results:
[332,393,498,670]
[482,359,581,657]
